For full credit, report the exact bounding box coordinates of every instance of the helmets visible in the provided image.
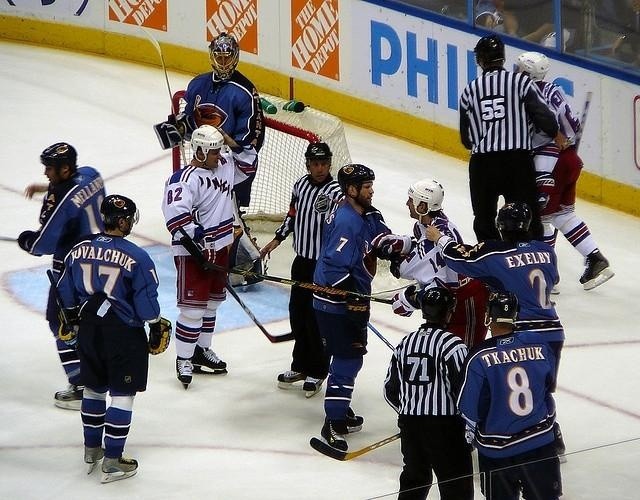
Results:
[305,142,332,160]
[191,124,224,163]
[421,287,458,318]
[407,178,444,212]
[474,35,505,61]
[515,52,550,80]
[41,142,76,166]
[338,164,375,185]
[100,195,139,225]
[497,202,533,232]
[486,289,519,324]
[209,32,239,81]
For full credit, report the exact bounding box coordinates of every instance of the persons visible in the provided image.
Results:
[153,32,268,289]
[459,36,570,246]
[424,201,566,464]
[57,195,164,475]
[260,141,346,398]
[372,179,488,351]
[21,141,114,409]
[160,127,262,384]
[312,163,402,453]
[453,0,639,68]
[456,290,563,500]
[383,287,474,500]
[512,51,617,284]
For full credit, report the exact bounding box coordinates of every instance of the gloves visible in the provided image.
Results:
[58,322,78,350]
[18,231,35,251]
[371,232,411,256]
[391,284,418,317]
[197,235,216,270]
[535,171,555,209]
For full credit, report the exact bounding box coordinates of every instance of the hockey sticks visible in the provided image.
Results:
[178,224,295,343]
[140,25,187,168]
[180,234,395,325]
[310,433,401,461]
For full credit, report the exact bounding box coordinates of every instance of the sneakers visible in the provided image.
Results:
[321,407,363,451]
[83,446,138,473]
[55,385,83,402]
[579,248,609,284]
[303,376,325,391]
[175,356,194,383]
[278,370,307,383]
[192,344,226,369]
